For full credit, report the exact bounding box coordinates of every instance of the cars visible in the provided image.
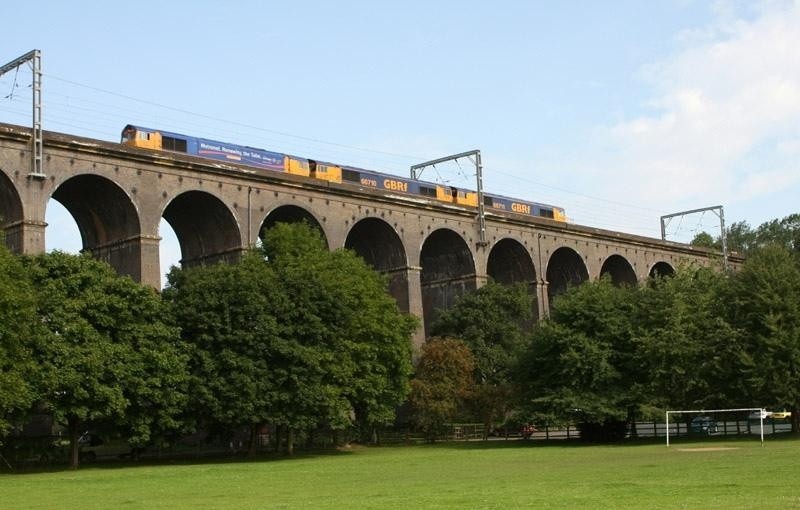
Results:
[747,406,792,421]
[690,416,718,436]
[492,422,538,438]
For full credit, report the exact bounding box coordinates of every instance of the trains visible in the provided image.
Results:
[119,122,568,227]
